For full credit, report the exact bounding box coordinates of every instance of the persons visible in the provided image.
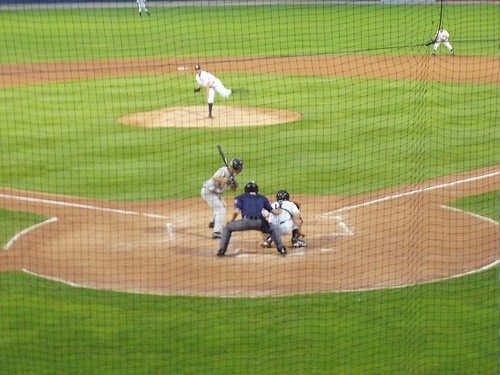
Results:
[200,158,243,239]
[194,65,248,119]
[260,190,307,247]
[136,0,151,18]
[215,182,287,257]
[430,26,455,56]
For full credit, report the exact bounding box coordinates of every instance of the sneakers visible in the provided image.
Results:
[212,232,222,239]
[292,240,307,247]
[280,248,287,254]
[217,250,224,256]
[209,222,214,228]
[261,240,270,248]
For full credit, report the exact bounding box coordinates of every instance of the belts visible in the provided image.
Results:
[244,215,262,219]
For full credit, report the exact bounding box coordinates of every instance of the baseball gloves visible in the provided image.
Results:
[194,88,200,92]
[294,202,299,209]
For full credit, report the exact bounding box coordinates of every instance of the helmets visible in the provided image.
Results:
[244,181,258,193]
[276,190,289,202]
[230,158,243,168]
[195,65,200,70]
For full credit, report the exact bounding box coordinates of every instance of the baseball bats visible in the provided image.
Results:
[217,146,237,187]
